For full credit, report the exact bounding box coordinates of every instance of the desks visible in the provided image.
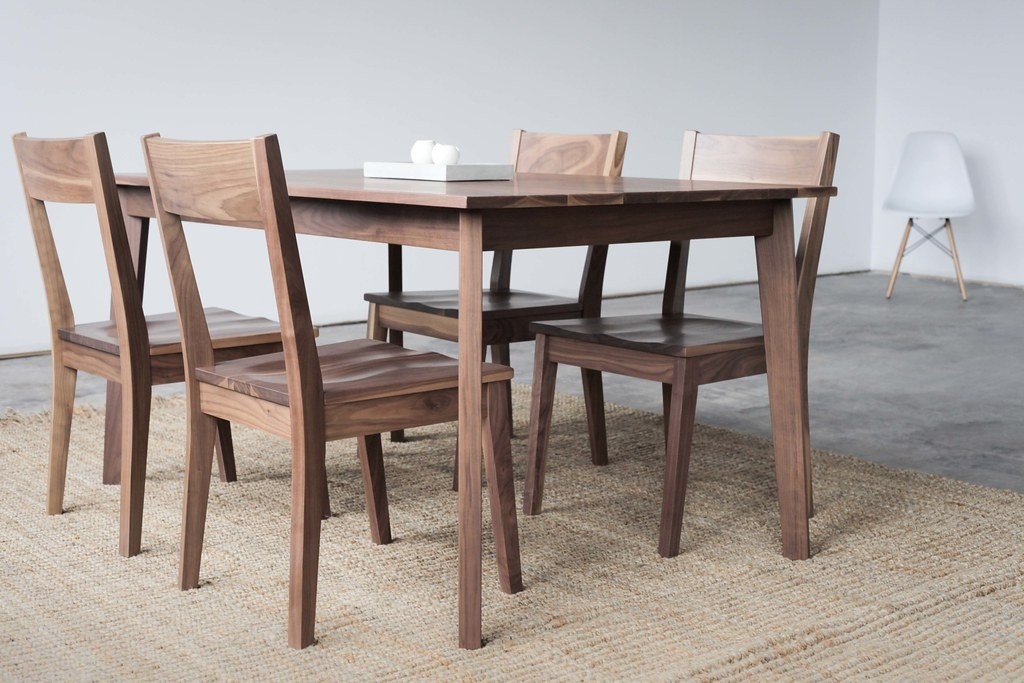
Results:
[101,169,838,650]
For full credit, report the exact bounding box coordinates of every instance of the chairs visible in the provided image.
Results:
[14,132,331,558]
[882,131,977,300]
[524,131,840,558]
[356,132,628,492]
[142,133,522,648]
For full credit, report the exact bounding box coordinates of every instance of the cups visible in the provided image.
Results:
[411,140,434,164]
[432,144,460,164]
